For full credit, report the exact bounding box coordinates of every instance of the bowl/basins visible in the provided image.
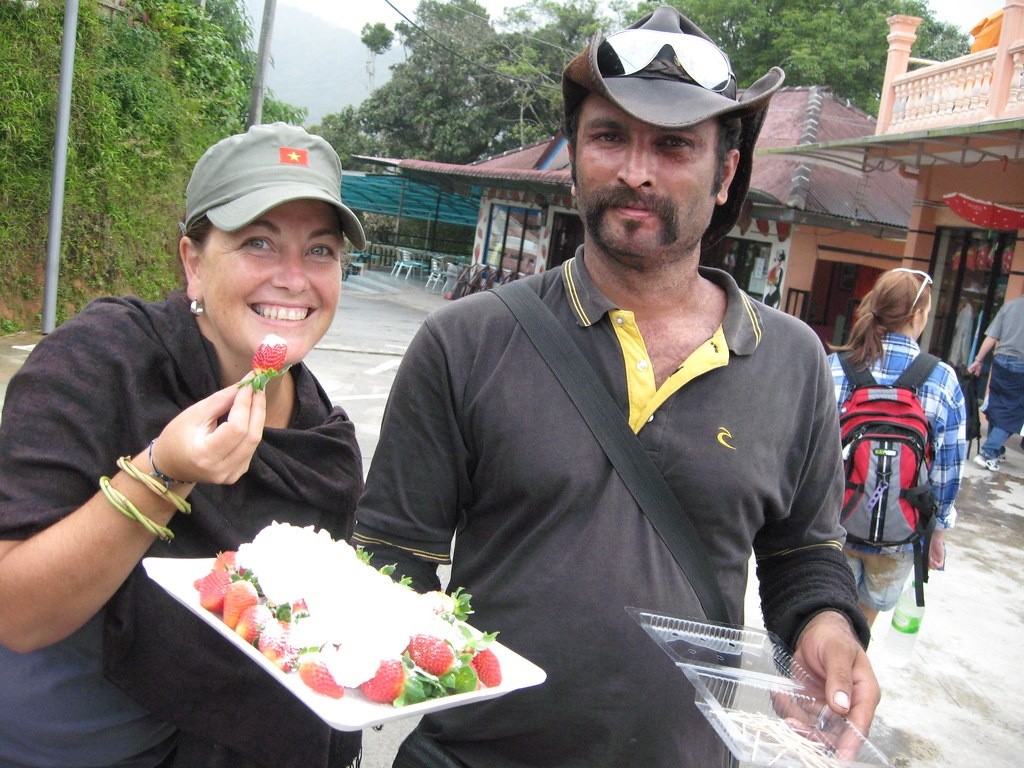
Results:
[623,604,896,768]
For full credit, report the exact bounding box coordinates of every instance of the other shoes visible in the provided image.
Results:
[998,454,1005,462]
[974,452,999,471]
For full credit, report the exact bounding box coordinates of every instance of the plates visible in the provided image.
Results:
[143,556,547,733]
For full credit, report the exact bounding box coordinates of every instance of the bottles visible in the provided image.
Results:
[885,581,924,668]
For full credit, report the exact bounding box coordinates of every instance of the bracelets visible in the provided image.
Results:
[974,356,984,363]
[975,356,984,363]
[99,436,197,544]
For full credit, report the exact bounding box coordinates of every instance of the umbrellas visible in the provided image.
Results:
[941,155,1024,239]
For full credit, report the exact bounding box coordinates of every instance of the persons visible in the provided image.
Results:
[826,269,967,632]
[968,294,1024,472]
[0,122,365,768]
[352,6,881,768]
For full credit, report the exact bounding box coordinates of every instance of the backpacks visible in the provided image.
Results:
[840,350,941,546]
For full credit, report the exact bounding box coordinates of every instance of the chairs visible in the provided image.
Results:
[348,240,381,268]
[392,248,448,291]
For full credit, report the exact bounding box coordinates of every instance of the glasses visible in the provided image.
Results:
[892,268,932,311]
[597,28,736,93]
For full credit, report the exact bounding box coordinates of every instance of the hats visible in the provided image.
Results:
[186,123,365,252]
[561,5,786,252]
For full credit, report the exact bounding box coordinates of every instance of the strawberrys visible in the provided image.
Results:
[195,550,500,701]
[254,335,289,373]
